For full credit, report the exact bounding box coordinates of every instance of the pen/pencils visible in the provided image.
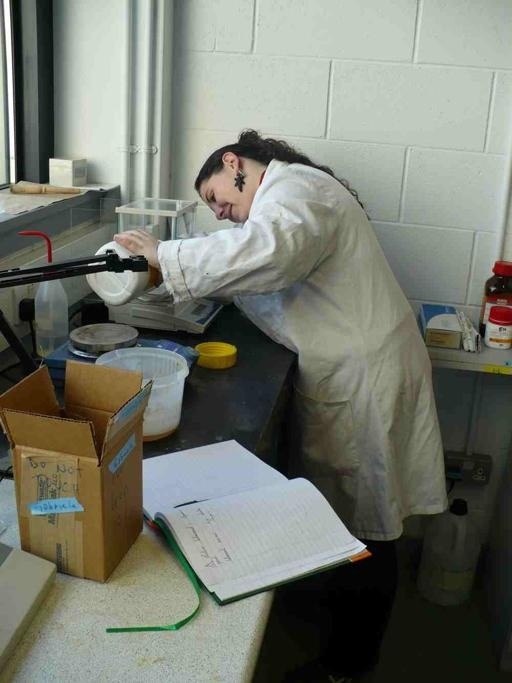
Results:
[174,498,209,509]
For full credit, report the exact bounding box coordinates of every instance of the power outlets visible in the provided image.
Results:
[445,450,492,487]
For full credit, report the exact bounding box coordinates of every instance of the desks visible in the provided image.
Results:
[0,300,298,462]
[427,325,511,377]
[0,478,276,683]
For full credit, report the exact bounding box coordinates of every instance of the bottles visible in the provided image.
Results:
[478,259,512,349]
[33,262,70,358]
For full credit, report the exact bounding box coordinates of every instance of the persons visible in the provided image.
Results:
[114,130,449,682]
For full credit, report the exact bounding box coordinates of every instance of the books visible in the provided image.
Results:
[140,438,371,606]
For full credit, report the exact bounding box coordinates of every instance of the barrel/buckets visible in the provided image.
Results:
[417,498,481,606]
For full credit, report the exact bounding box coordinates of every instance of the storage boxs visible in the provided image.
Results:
[0,360,153,584]
